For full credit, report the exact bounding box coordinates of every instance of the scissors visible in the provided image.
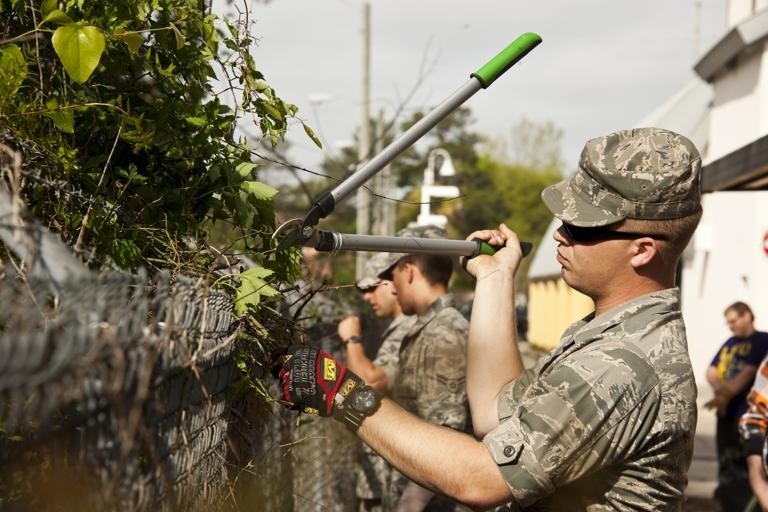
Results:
[272,31,542,258]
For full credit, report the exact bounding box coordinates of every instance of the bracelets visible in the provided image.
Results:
[341,335,363,347]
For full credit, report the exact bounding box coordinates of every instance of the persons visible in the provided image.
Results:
[261,118,704,511]
[697,300,768,512]
[359,222,475,512]
[328,252,420,512]
[727,353,768,512]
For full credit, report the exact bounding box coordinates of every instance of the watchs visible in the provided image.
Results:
[333,383,388,430]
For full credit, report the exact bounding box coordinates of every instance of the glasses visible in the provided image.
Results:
[563,224,666,244]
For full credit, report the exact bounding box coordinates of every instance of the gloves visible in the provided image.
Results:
[269,346,364,421]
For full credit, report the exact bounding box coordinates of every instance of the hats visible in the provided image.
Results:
[541,128,701,228]
[355,224,450,290]
